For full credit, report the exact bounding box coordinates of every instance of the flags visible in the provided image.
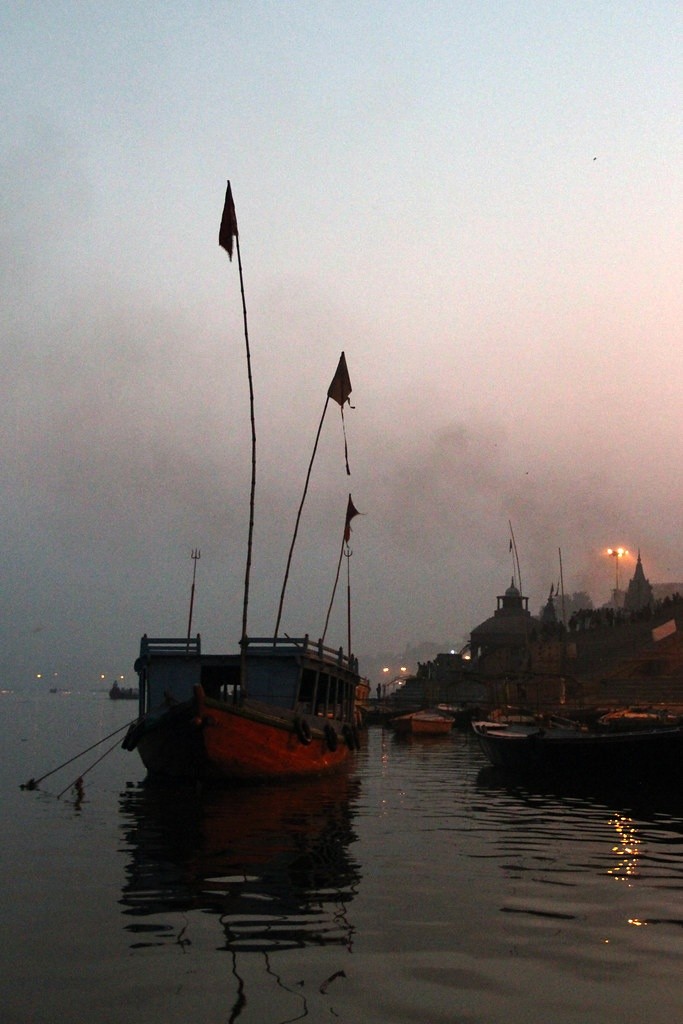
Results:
[343,495,361,544]
[555,581,559,597]
[509,538,512,551]
[327,351,353,406]
[218,180,239,262]
[550,582,554,596]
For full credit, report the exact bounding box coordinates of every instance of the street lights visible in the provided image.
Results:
[607,547,628,590]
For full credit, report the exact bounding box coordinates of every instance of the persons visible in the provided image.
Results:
[376,683,381,698]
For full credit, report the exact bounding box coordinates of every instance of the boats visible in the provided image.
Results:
[469,718,683,773]
[122,177,368,775]
[389,712,417,732]
[410,710,456,735]
